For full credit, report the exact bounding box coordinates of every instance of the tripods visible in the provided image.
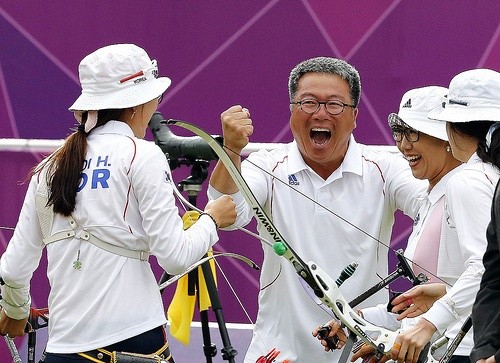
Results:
[158,162,237,363]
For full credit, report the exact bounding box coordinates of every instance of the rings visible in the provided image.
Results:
[393,343,401,351]
[407,298,412,305]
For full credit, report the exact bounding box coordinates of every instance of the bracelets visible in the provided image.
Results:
[198,212,219,230]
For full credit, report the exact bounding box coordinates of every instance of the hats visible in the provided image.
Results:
[388,86,450,142]
[426,69,500,122]
[68,43,171,111]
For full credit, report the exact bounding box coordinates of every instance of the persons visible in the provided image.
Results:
[311,68,500,363]
[0,44,237,363]
[206,56,430,363]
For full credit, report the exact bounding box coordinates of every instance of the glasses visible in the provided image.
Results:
[157,93,164,104]
[289,98,356,115]
[391,128,423,141]
